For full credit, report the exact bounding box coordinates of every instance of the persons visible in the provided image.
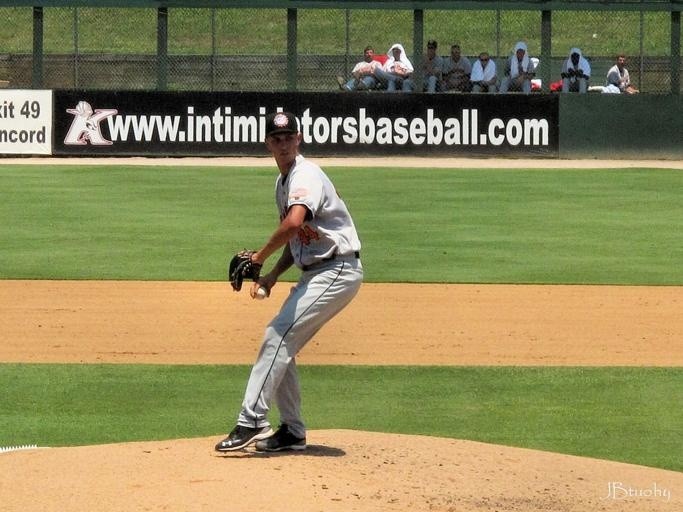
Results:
[214,109,365,453]
[335,39,639,94]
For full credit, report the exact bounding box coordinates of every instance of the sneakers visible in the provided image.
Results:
[255,424,305,452]
[215,424,273,451]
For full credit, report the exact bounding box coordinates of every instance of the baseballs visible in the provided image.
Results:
[255,287,267,300]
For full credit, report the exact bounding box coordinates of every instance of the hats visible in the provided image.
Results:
[265,112,297,137]
[427,39,437,48]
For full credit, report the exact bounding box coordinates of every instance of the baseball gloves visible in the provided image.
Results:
[228,248,262,292]
